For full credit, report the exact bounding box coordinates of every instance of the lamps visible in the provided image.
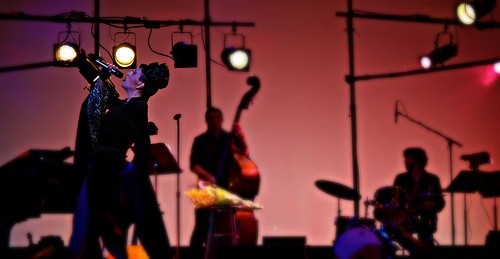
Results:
[170,24,198,68]
[456,0,497,26]
[112,24,137,70]
[53,22,81,68]
[420,23,458,70]
[221,26,250,73]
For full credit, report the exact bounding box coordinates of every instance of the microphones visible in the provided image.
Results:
[394,102,398,122]
[88,54,124,79]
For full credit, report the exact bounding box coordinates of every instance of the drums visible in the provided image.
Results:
[334,225,396,259]
[405,203,436,233]
[334,215,375,245]
[372,186,410,225]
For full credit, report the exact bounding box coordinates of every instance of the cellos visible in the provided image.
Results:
[198,74,262,258]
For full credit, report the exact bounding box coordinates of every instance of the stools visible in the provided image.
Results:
[202,206,240,259]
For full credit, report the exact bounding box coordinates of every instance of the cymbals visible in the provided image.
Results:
[315,180,361,201]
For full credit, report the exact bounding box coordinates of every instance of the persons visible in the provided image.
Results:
[190,107,250,250]
[68,63,171,259]
[395,147,445,246]
